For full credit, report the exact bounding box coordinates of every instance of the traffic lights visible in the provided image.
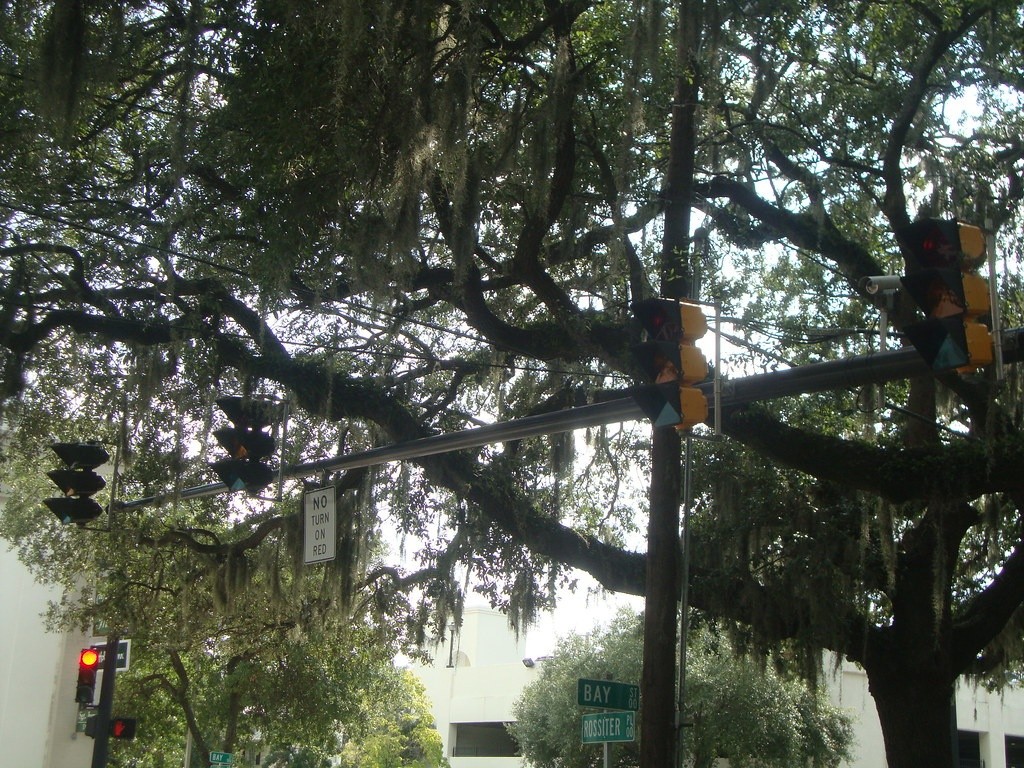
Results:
[627,296,709,431]
[75,648,98,703]
[41,442,111,527]
[208,394,276,493]
[908,215,994,377]
[110,717,136,741]
